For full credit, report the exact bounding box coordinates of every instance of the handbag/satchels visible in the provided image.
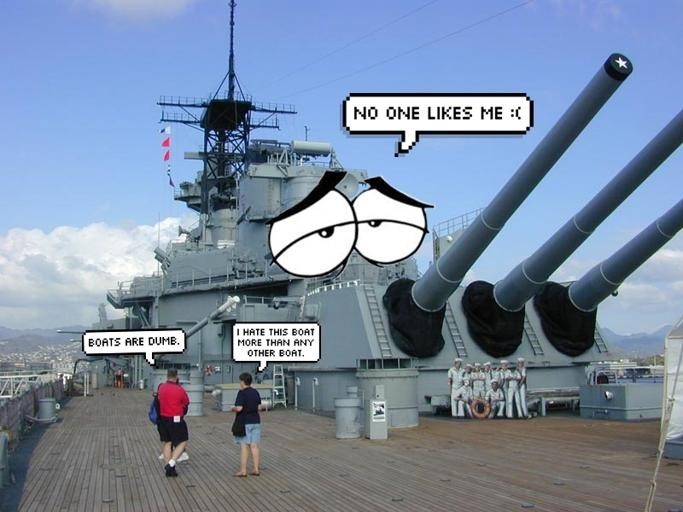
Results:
[231,414,246,437]
[148,392,160,425]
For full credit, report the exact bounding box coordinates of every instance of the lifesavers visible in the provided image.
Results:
[469,398,491,419]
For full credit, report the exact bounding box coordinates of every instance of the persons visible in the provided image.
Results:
[113,367,124,387]
[445,356,530,419]
[155,378,188,463]
[229,372,262,476]
[153,367,189,477]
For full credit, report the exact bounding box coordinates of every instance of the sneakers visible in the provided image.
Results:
[159,453,164,460]
[176,452,189,462]
[164,464,178,477]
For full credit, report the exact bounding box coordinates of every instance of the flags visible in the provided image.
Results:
[159,127,171,134]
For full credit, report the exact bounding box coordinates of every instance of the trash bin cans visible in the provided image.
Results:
[38,398,56,420]
[333,397,361,439]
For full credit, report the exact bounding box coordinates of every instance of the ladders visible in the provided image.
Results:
[272,364,287,408]
